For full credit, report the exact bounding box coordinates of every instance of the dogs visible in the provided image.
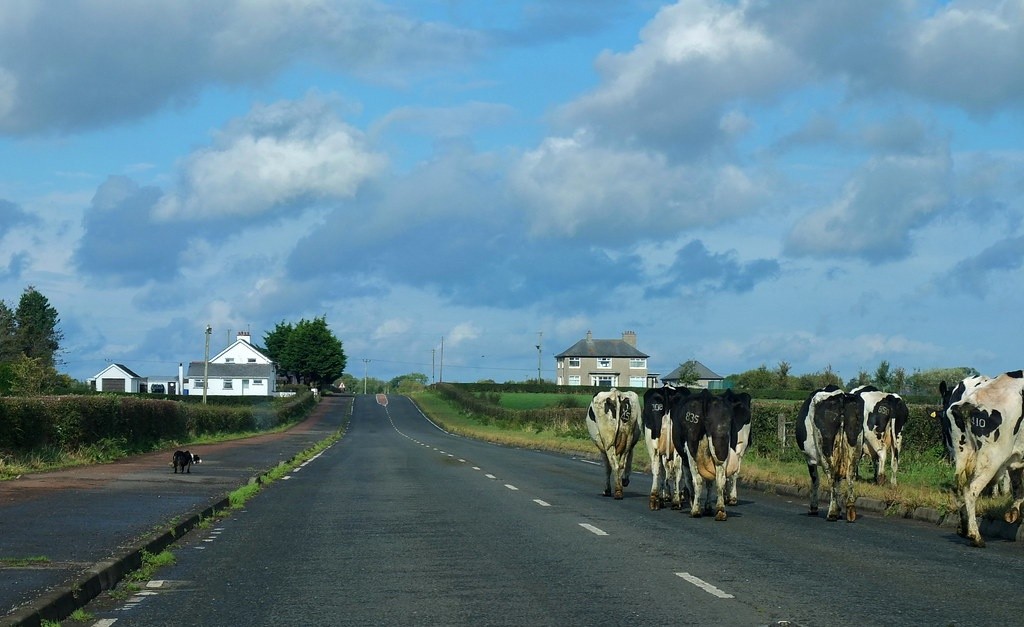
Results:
[168,451,202,473]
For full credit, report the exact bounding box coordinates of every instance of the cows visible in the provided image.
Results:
[926,370,1024,548]
[585,388,641,500]
[793,384,909,523]
[642,383,753,521]
